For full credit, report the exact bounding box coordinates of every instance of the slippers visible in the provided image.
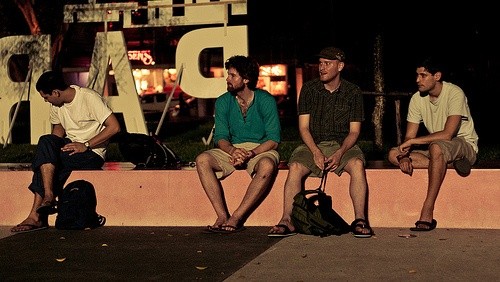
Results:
[453,156,471,176]
[35,197,58,214]
[205,224,221,233]
[10,222,48,233]
[216,224,247,233]
[266,223,298,237]
[411,218,437,232]
[350,217,374,237]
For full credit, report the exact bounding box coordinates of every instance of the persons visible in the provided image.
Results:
[387,59,478,230]
[196,56,284,232]
[10,71,121,233]
[268,48,373,237]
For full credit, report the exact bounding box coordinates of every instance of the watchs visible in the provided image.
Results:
[85,141,91,150]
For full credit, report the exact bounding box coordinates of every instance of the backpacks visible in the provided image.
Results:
[292,185,349,237]
[120,133,182,170]
[53,179,107,231]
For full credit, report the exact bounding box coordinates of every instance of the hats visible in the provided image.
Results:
[318,47,345,62]
